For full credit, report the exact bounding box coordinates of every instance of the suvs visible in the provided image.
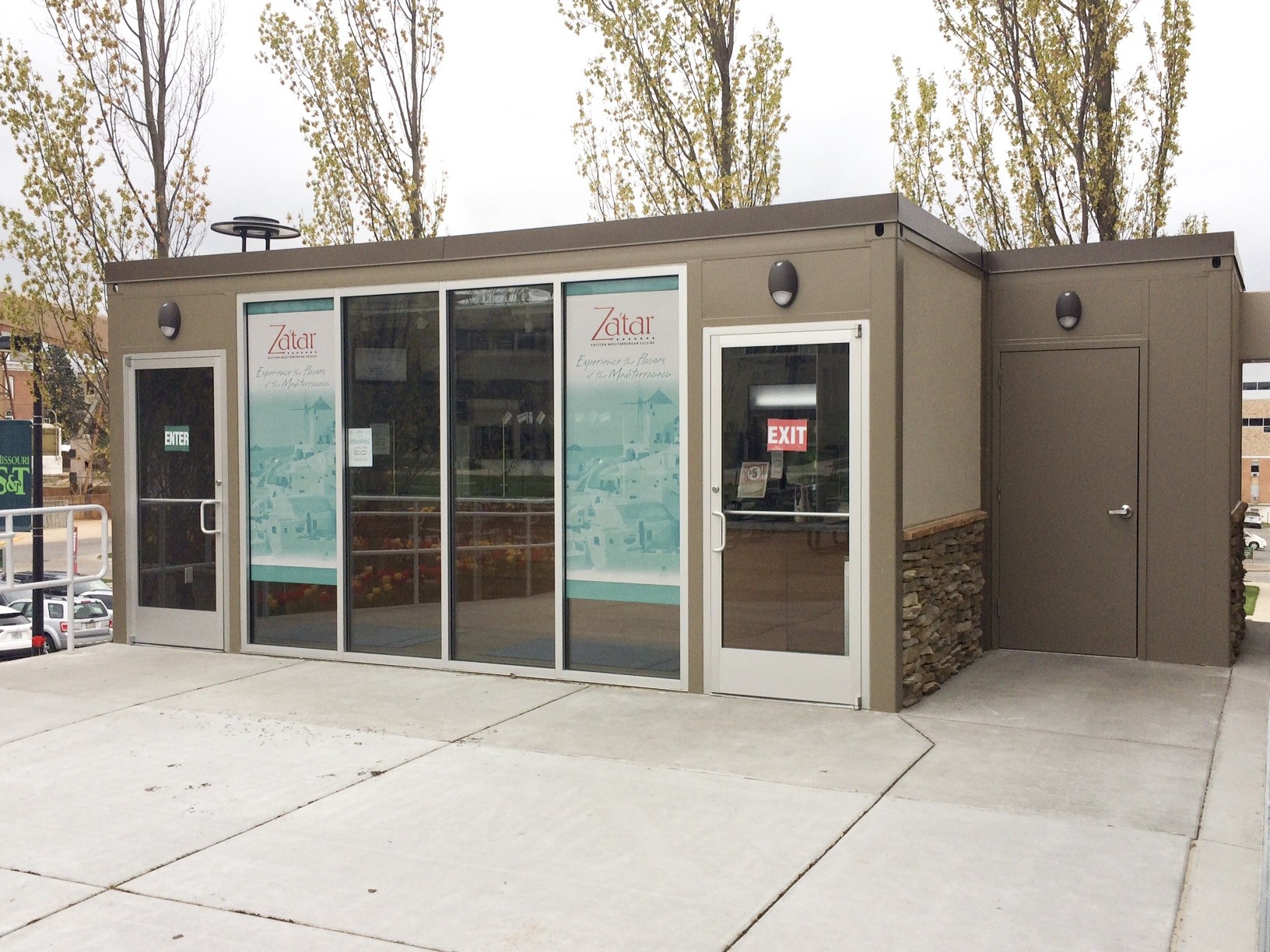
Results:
[0,604,48,663]
[0,570,113,611]
[6,595,114,653]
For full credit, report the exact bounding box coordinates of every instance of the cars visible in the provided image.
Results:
[1243,530,1267,550]
[0,580,46,607]
[1243,510,1264,529]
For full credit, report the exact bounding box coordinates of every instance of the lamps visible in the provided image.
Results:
[1056,290,1081,330]
[158,301,181,339]
[767,260,797,307]
